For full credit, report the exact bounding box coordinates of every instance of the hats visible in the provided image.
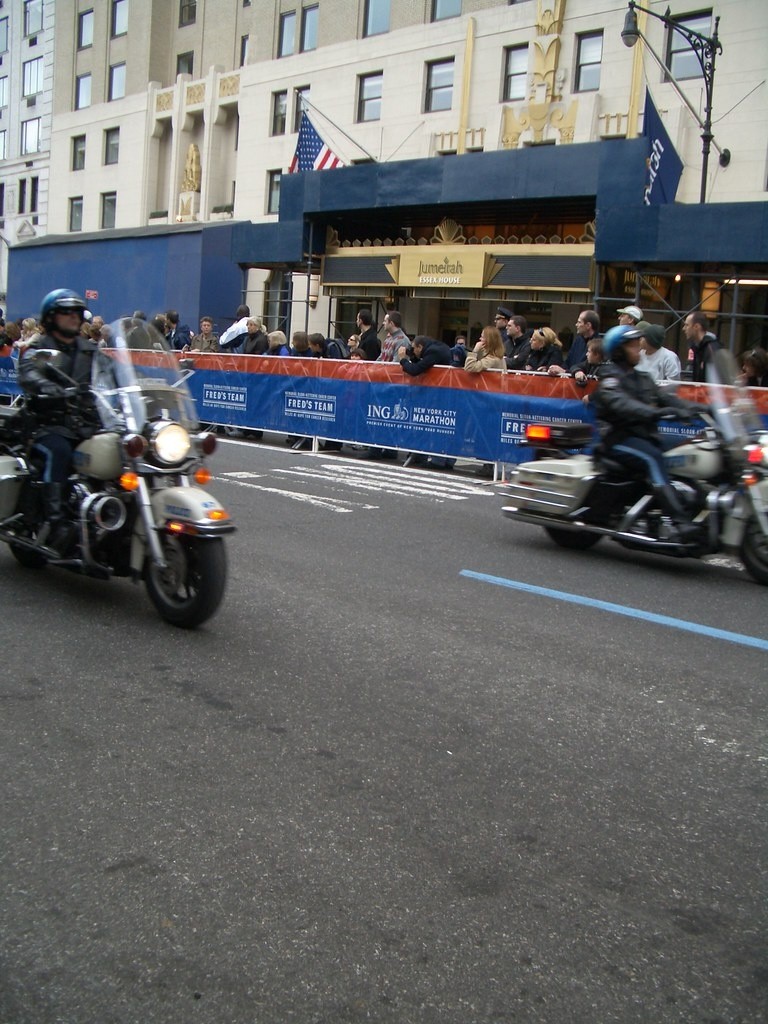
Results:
[616,305,644,321]
[644,325,665,345]
[494,306,513,320]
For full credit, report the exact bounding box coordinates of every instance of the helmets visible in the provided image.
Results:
[38,288,88,328]
[601,325,646,361]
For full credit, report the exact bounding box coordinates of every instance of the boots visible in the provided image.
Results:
[39,480,84,555]
[652,485,705,544]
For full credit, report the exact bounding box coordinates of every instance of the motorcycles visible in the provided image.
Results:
[494,348,768,590]
[0,314,237,630]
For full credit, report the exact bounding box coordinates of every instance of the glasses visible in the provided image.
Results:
[348,337,356,342]
[56,307,82,315]
[507,324,515,328]
[246,323,256,327]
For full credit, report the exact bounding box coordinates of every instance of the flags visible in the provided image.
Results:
[289,111,347,173]
[642,84,684,204]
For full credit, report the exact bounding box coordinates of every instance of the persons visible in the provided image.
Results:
[0,305,768,475]
[18,287,134,568]
[593,325,715,538]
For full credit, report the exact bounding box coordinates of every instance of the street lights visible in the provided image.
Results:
[620,0,722,206]
